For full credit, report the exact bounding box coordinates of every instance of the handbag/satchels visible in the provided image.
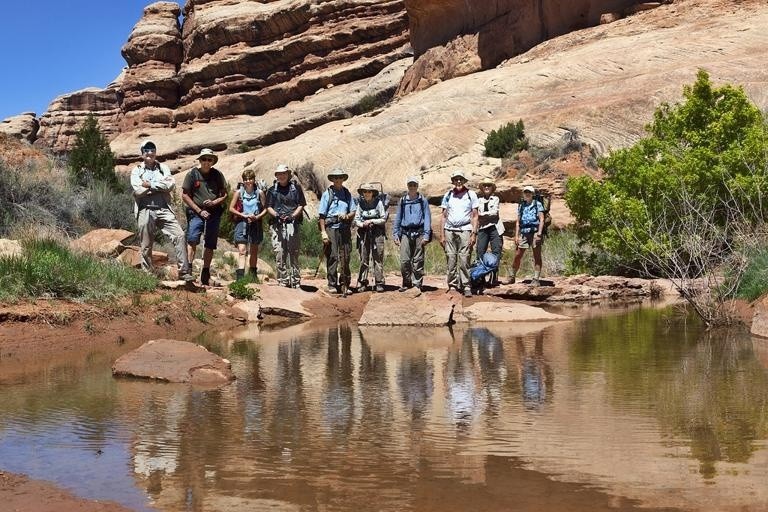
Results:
[468,252,500,287]
[230,199,243,222]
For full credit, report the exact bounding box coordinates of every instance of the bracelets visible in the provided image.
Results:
[198,209,204,215]
[536,232,542,237]
[471,231,477,235]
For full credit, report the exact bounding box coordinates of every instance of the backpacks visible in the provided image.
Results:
[520,194,552,235]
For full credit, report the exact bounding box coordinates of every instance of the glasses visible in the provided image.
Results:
[482,184,492,187]
[363,190,371,194]
[201,157,213,162]
[143,148,156,152]
[454,178,463,181]
[334,176,345,180]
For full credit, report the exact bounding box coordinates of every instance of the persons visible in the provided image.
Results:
[226,169,269,283]
[438,169,480,298]
[314,168,356,296]
[129,139,196,283]
[262,165,307,289]
[390,176,432,293]
[354,182,388,292]
[472,177,506,265]
[181,150,228,285]
[499,185,543,288]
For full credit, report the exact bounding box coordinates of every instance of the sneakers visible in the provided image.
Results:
[357,286,368,293]
[329,287,338,293]
[178,274,196,282]
[507,276,515,284]
[464,288,472,297]
[341,286,352,295]
[399,287,408,292]
[376,285,385,292]
[531,278,540,286]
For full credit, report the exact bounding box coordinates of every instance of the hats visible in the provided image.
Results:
[140,140,156,152]
[274,165,292,181]
[328,168,348,181]
[196,148,218,166]
[451,171,469,184]
[357,184,380,196]
[523,186,535,193]
[407,176,418,184]
[479,178,496,192]
